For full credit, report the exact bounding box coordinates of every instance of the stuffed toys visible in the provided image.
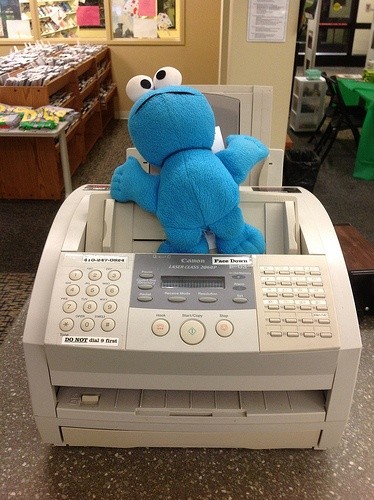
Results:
[111,66,268,254]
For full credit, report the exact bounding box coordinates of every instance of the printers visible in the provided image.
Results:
[18,86,362,452]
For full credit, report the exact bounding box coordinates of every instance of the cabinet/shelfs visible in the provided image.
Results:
[289,73,327,133]
[0,0,118,202]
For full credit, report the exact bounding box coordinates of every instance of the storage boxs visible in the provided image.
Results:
[332,222,374,316]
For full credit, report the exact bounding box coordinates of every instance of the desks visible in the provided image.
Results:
[334,73,374,184]
[0,294,374,500]
[0,119,73,198]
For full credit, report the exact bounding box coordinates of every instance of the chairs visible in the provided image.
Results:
[307,72,368,169]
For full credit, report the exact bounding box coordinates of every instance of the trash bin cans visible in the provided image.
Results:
[285,149,320,195]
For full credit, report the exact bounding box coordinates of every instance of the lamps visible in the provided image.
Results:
[304,0,318,19]
[332,0,346,11]
